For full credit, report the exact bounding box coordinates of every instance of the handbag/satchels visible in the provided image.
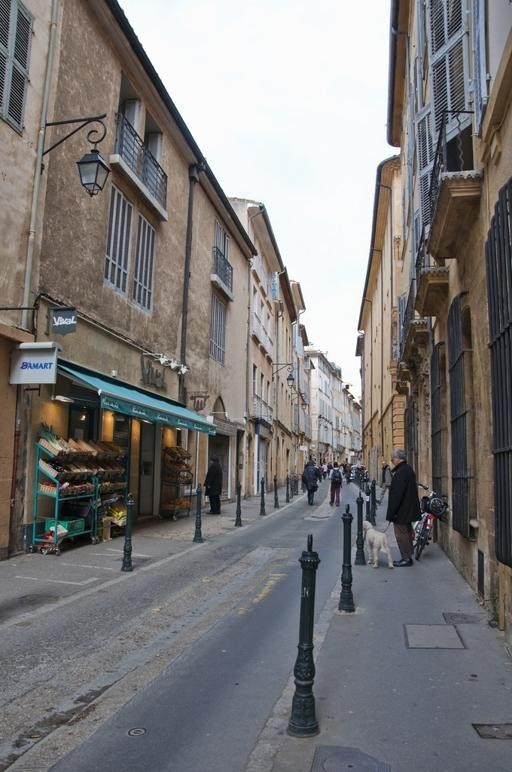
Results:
[307,480,318,492]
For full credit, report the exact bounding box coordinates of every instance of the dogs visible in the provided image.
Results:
[362,521,394,569]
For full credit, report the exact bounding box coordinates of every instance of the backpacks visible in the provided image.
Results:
[331,467,341,488]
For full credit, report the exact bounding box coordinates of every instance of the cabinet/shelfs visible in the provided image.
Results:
[159,444,194,520]
[29,433,127,555]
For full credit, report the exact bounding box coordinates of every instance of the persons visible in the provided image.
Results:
[300,456,355,506]
[386,447,422,567]
[204,455,224,515]
[376,461,393,504]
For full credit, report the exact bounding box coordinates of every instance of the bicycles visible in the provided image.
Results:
[412,482,448,559]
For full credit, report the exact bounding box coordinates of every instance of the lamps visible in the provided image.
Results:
[49,379,75,406]
[143,352,188,377]
[272,361,295,391]
[40,111,113,200]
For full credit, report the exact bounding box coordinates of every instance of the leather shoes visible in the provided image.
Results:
[393,558,413,567]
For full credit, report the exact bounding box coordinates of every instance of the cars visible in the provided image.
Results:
[349,464,369,482]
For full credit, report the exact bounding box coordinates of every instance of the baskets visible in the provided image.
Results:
[38,435,128,538]
[162,446,193,512]
